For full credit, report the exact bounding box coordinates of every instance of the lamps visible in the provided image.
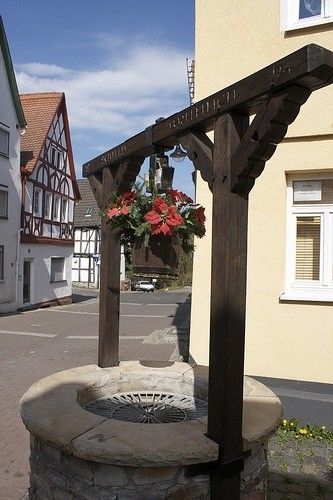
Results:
[170,142,189,163]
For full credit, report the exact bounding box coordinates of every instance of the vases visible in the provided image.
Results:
[132,232,183,281]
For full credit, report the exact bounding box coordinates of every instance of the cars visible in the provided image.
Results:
[136,280,155,293]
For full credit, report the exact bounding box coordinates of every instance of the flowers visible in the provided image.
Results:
[96,173,206,256]
